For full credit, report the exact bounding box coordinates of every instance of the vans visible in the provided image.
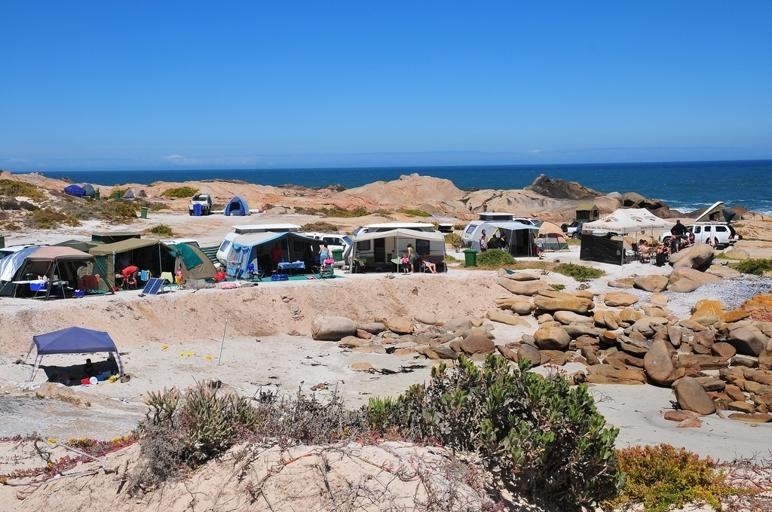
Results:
[660,225,738,247]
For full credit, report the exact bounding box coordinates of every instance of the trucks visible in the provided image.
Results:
[188,193,211,215]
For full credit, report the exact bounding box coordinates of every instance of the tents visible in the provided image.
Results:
[65,183,95,199]
[0,230,219,298]
[224,195,249,216]
[227,232,323,279]
[533,222,571,252]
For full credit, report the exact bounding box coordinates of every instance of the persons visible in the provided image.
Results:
[480,230,508,252]
[82,359,93,379]
[536,234,545,259]
[401,243,437,274]
[318,242,329,262]
[636,220,694,266]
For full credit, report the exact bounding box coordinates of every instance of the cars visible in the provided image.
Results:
[567,219,580,238]
[514,217,540,226]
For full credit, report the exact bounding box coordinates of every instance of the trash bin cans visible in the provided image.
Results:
[141,207,148,218]
[115,192,120,199]
[95,192,100,200]
[463,249,478,268]
